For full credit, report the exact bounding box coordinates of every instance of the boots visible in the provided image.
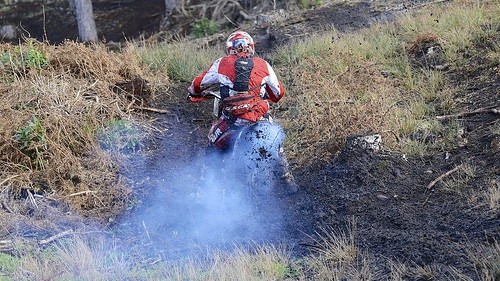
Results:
[277,172,298,195]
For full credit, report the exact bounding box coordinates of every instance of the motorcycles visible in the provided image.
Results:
[186,83,276,227]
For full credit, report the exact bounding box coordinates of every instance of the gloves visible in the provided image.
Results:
[262,92,270,101]
[186,91,203,103]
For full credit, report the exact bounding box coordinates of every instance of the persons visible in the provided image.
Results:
[185,30,298,208]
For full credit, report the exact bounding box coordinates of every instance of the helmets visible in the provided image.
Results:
[225,30,255,58]
